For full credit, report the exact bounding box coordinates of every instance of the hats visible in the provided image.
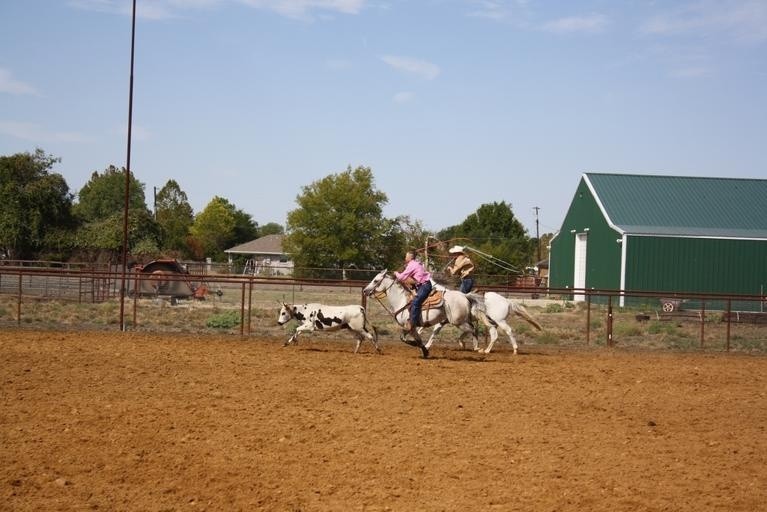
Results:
[449,245,464,253]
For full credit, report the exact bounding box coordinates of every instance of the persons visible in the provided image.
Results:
[393,249,432,332]
[445,245,474,299]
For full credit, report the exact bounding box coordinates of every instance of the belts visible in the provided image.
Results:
[416,280,430,288]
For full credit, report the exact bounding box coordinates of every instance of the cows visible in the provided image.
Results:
[277,300,384,354]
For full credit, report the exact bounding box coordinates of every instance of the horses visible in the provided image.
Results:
[416,276,542,356]
[363,267,488,355]
